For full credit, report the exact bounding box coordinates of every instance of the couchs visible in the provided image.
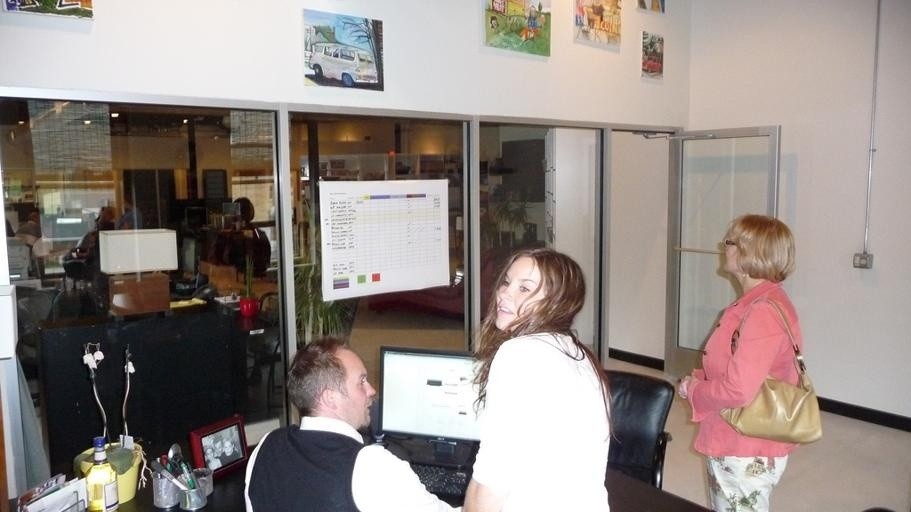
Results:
[368,244,546,323]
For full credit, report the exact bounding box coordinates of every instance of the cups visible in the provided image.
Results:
[194,468,213,495]
[151,470,179,509]
[177,474,208,511]
[240,296,258,318]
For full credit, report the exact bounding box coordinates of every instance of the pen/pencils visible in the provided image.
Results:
[150,455,203,504]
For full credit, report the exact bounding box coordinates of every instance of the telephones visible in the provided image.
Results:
[192,284,216,299]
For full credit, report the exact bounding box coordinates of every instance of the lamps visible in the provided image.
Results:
[99,227,178,316]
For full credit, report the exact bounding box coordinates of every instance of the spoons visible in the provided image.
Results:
[167,442,182,463]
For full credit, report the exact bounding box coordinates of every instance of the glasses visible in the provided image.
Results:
[724,236,737,246]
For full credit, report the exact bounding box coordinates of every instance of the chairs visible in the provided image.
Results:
[604,370,674,490]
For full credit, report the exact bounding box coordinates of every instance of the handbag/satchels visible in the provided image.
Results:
[720,372,824,442]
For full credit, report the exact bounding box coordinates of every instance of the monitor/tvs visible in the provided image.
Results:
[380,346,487,469]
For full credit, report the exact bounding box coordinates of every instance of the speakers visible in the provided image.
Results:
[368,400,386,439]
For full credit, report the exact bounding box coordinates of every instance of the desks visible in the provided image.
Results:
[122,441,727,511]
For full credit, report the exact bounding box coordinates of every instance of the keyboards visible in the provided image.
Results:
[409,463,471,500]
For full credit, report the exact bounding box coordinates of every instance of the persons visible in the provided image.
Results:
[244,336,455,512]
[16,212,41,238]
[462,247,610,512]
[676,214,802,512]
[96,206,115,231]
[205,438,241,470]
[206,198,270,278]
[115,193,143,229]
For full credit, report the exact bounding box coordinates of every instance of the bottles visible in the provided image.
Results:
[86,437,119,512]
